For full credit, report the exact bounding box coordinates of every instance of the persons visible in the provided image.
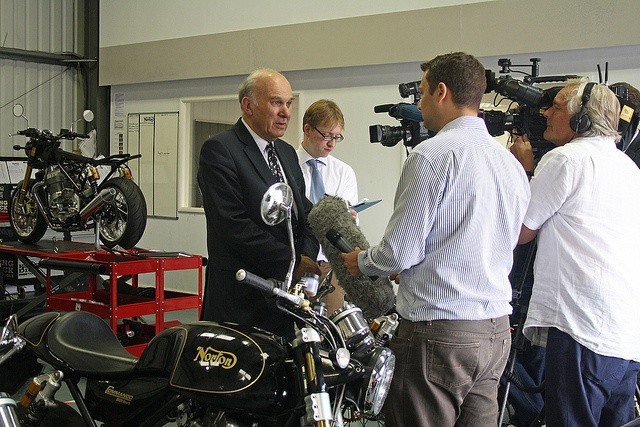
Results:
[338,52,532,425]
[297,98,358,317]
[510,76,640,425]
[197,67,319,345]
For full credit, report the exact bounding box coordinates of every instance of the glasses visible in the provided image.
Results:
[311,124,344,142]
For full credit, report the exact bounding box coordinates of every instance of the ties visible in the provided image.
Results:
[307,159,325,204]
[266,144,298,251]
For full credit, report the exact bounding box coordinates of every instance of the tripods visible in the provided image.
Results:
[494,230,548,426]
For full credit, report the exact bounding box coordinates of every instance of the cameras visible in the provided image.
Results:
[484,56,640,148]
[368,80,508,148]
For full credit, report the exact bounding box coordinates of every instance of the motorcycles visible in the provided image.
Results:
[8,103,147,251]
[2,182,399,427]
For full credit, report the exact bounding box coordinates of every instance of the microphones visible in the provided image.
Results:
[325,227,380,281]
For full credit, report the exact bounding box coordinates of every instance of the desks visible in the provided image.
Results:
[0,241,203,357]
